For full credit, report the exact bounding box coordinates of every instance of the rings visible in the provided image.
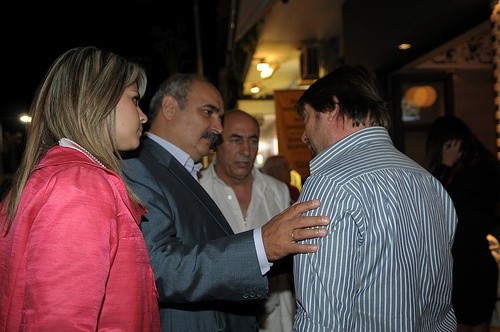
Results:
[291,230,296,240]
[451,143,455,146]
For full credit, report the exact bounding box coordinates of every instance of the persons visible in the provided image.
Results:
[0,46,162,332]
[115,71,330,332]
[426,115,500,332]
[201,108,298,332]
[289,62,460,331]
